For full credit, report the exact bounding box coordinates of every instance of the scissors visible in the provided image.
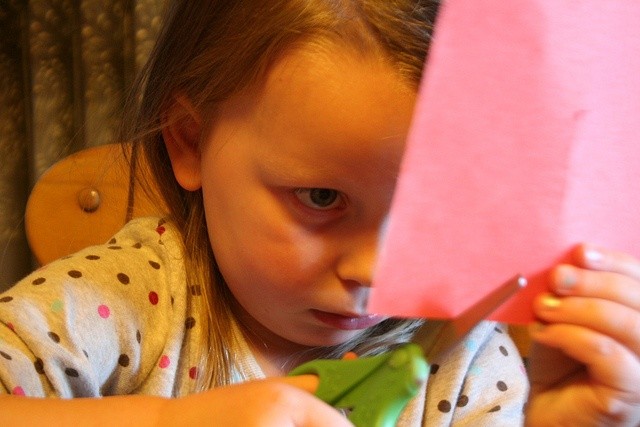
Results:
[287,273,529,426]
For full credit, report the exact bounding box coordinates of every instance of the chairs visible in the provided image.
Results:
[25,140,171,265]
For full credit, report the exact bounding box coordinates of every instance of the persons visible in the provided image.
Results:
[0,0,638,426]
